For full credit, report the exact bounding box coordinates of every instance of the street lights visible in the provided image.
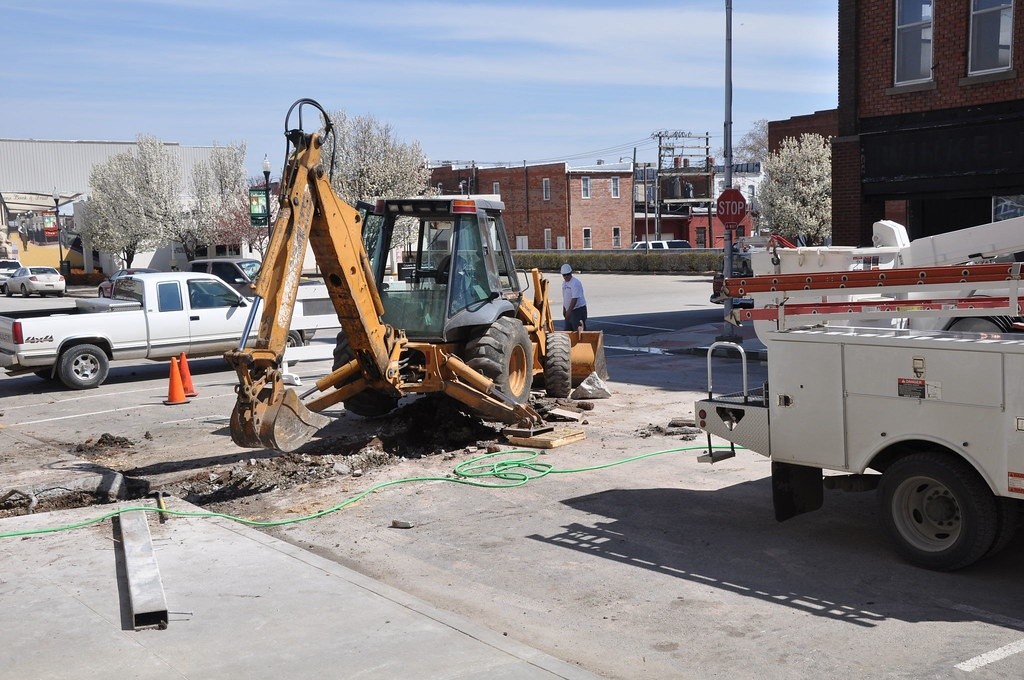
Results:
[261,154,273,234]
[52,184,66,273]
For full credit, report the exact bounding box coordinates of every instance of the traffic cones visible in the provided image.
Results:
[177,350,198,400]
[161,357,191,407]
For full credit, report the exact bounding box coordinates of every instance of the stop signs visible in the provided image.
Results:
[718,189,751,233]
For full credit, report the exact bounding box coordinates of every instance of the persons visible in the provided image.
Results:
[17,221,28,251]
[561,264,587,331]
[0,225,16,260]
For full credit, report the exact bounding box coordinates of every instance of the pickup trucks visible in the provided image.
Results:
[0,270,317,389]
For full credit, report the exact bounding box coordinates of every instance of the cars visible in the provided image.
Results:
[4,263,70,299]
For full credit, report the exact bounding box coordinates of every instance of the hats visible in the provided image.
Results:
[561,264,572,274]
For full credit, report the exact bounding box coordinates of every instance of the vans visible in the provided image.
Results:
[186,256,268,297]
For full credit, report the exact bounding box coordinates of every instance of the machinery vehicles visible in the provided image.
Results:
[222,98,612,454]
[694,213,1020,570]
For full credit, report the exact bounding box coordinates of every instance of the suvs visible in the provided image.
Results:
[0,259,22,293]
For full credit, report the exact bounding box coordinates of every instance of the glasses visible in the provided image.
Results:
[563,273,571,276]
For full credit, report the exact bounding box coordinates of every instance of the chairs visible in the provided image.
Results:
[421,254,467,312]
